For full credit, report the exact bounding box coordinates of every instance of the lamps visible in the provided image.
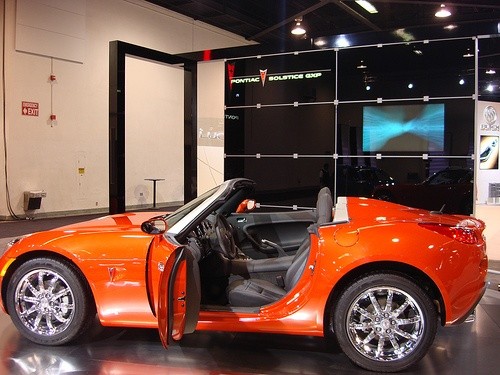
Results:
[435,4,452,18]
[291,15,306,35]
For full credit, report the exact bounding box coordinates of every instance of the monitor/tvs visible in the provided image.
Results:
[363,103,445,153]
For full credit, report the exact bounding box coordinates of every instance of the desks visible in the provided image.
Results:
[144,178,165,210]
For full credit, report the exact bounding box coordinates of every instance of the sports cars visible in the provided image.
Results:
[1,177,489,375]
[370,166,473,215]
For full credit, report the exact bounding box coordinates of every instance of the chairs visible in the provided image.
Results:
[227,187,333,307]
